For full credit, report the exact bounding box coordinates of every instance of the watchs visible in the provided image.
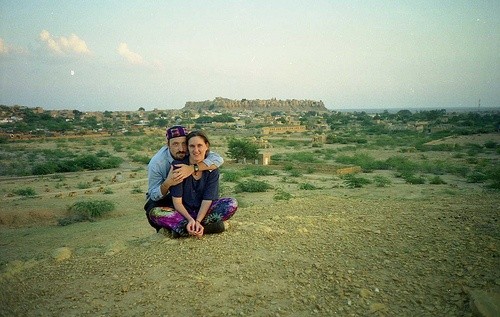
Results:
[193,163,199,172]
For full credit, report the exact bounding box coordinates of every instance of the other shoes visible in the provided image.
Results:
[204,220,229,234]
[171,230,179,239]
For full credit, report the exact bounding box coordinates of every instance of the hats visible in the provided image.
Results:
[166,125,188,139]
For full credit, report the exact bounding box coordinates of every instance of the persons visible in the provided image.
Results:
[143,125,225,232]
[149,130,238,238]
